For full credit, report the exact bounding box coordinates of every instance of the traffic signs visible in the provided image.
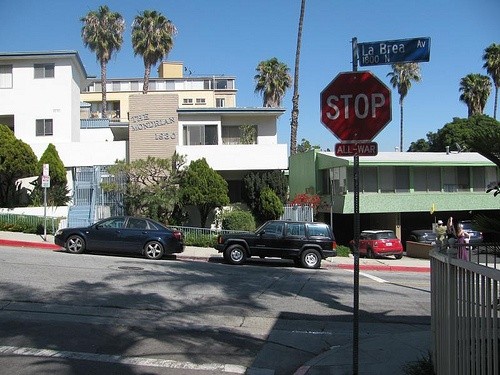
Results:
[358,36,431,66]
[335,141,378,156]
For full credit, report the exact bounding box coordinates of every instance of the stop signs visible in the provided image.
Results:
[320,71,392,142]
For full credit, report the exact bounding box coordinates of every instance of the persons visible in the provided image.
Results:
[455,222,471,263]
[447,217,457,238]
[433,219,448,244]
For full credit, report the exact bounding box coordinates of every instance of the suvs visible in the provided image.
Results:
[457,220,484,250]
[214,219,337,268]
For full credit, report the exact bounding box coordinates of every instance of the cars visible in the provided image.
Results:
[350,230,403,259]
[54,215,184,259]
